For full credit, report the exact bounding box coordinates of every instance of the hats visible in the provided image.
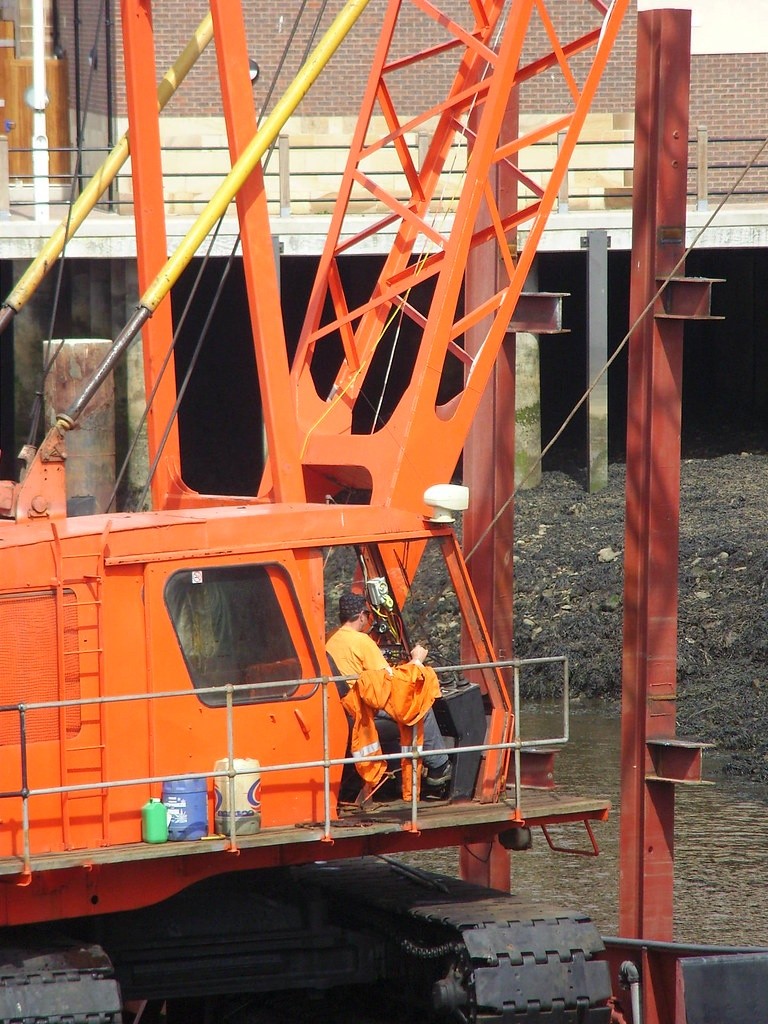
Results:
[339,593,369,613]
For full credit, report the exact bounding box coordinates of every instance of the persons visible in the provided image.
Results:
[325,594,453,787]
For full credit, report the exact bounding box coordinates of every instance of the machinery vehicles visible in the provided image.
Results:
[0,0,638,1024]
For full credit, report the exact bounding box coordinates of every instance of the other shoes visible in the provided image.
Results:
[426,760,455,786]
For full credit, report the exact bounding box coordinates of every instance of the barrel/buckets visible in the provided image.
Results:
[141,797,168,843]
[213,758,262,835]
[162,777,208,841]
